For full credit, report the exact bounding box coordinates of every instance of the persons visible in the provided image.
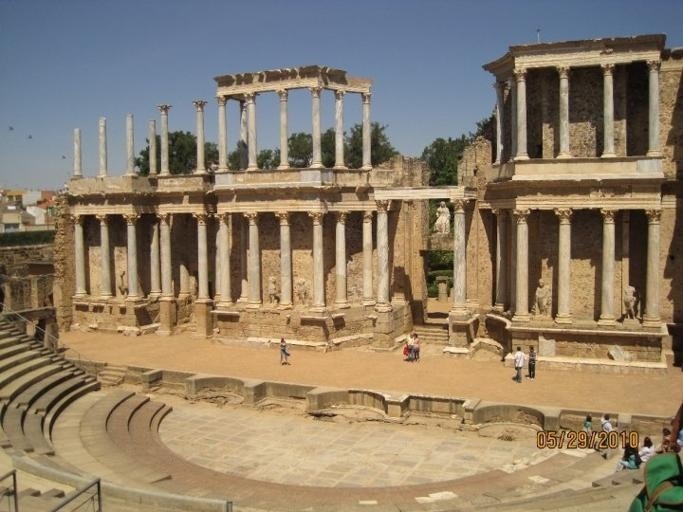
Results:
[433,201,451,234]
[534,279,551,315]
[412,334,421,361]
[279,337,291,366]
[513,346,526,383]
[267,273,281,305]
[527,345,537,381]
[582,399,683,512]
[297,278,308,304]
[623,283,637,320]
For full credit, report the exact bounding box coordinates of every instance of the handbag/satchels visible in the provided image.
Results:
[628,452,683,512]
